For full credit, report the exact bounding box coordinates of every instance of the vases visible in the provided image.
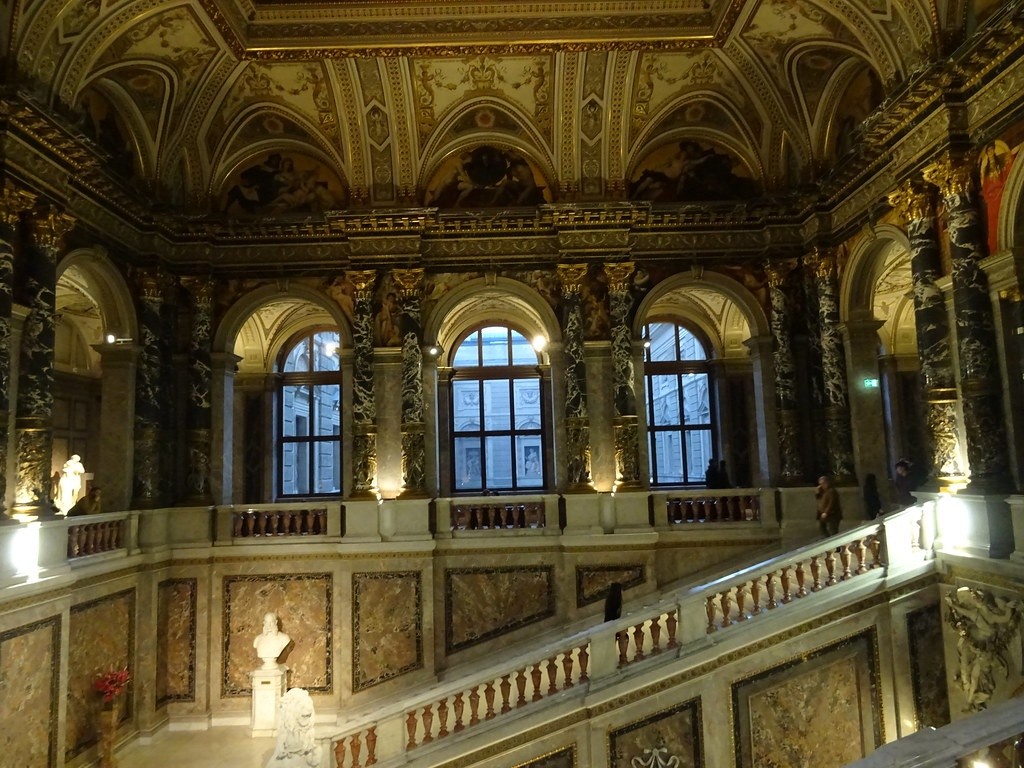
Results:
[103,695,119,711]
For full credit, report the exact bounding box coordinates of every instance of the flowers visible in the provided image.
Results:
[91,660,135,703]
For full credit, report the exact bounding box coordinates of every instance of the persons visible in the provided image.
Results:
[815,458,915,552]
[67,488,101,517]
[605,582,623,639]
[59,455,85,506]
[945,587,1016,712]
[254,613,291,666]
[706,458,730,490]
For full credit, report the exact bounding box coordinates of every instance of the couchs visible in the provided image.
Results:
[274,496,342,530]
[651,485,728,519]
[453,490,546,523]
